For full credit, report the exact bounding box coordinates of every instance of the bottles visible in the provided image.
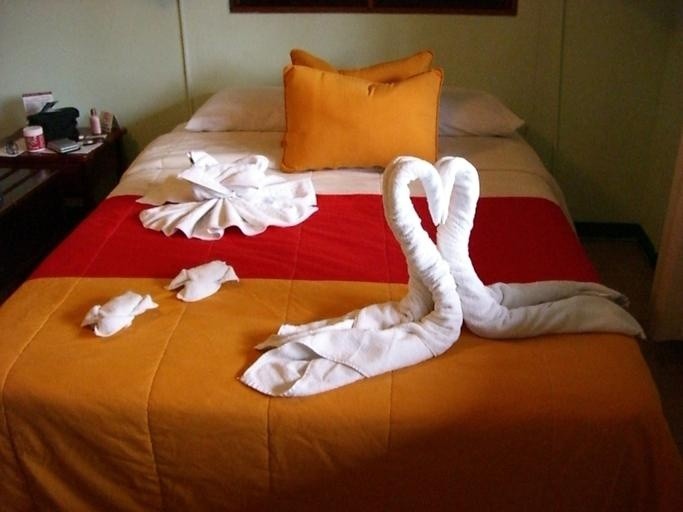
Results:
[23,126,46,153]
[90,108,101,135]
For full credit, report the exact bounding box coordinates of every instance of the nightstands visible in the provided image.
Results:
[0,127,128,241]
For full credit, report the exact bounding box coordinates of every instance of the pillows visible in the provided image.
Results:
[279,65,443,170]
[187,89,286,136]
[279,50,433,150]
[438,88,523,137]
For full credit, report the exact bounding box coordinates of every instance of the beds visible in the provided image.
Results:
[0,120,683,512]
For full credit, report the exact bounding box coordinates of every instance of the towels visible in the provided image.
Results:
[434,156,648,342]
[78,290,159,337]
[139,167,319,241]
[164,259,240,302]
[135,149,288,208]
[236,156,463,400]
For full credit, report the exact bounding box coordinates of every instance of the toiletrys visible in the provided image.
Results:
[88,107,101,135]
